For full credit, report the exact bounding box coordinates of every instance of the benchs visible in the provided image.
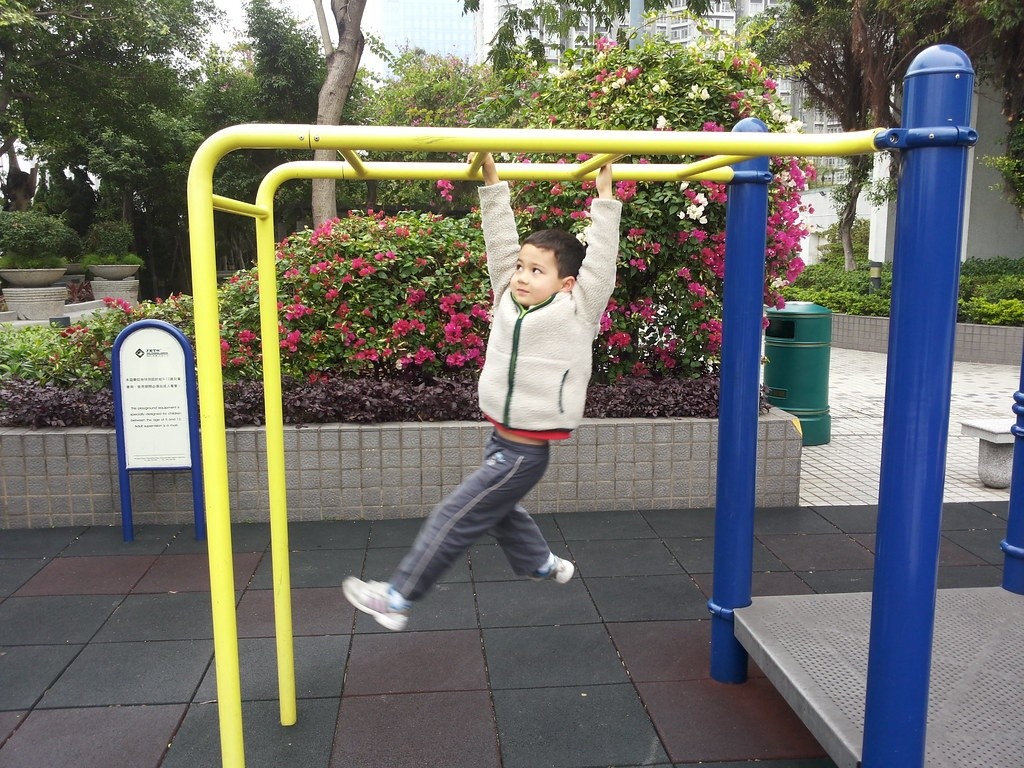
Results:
[956,418,1017,488]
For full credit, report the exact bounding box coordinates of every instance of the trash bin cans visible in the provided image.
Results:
[763,301,833,447]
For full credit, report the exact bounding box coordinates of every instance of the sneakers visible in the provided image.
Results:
[526,555,574,584]
[343,577,414,631]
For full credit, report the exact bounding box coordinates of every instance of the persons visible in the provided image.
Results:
[339,147,625,635]
